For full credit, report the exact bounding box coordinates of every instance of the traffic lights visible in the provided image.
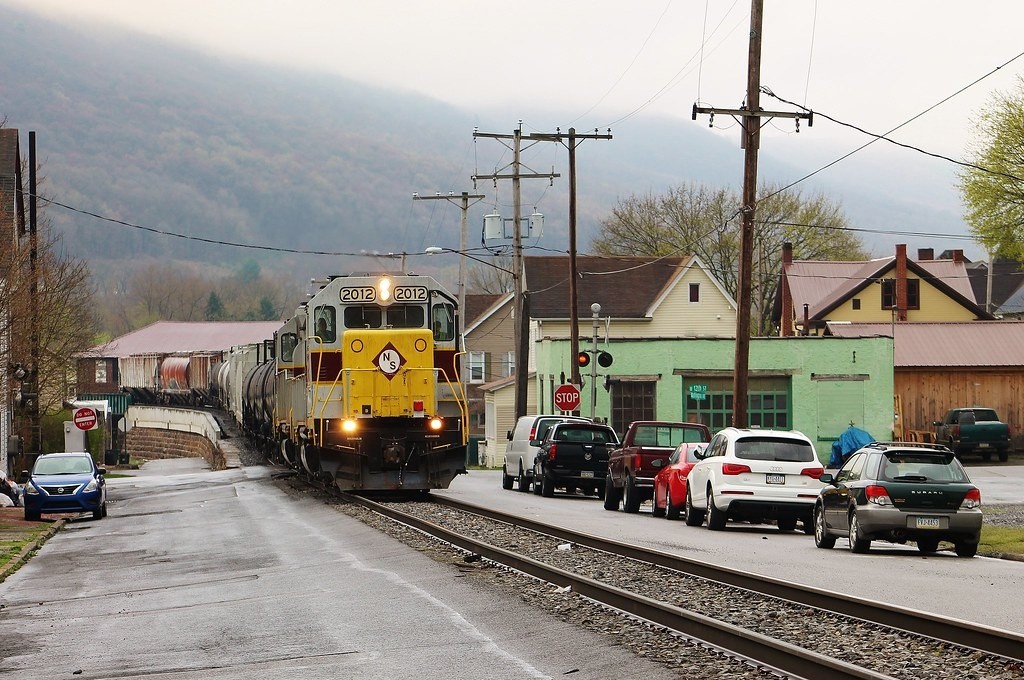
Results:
[578,352,590,367]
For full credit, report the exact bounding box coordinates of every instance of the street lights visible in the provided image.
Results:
[425,247,525,414]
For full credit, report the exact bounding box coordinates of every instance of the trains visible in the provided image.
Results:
[117,271,469,496]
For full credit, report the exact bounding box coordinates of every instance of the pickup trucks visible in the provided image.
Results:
[604,422,711,513]
[934,409,1012,464]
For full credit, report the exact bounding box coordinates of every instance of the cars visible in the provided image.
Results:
[651,439,715,519]
[503,415,594,491]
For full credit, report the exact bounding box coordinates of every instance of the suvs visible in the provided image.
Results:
[21,450,107,520]
[685,429,827,534]
[532,422,620,497]
[808,443,984,556]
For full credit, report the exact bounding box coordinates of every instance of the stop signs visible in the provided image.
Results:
[73,409,99,428]
[554,384,581,411]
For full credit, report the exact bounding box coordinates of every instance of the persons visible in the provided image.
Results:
[316,318,333,341]
[434,321,448,339]
